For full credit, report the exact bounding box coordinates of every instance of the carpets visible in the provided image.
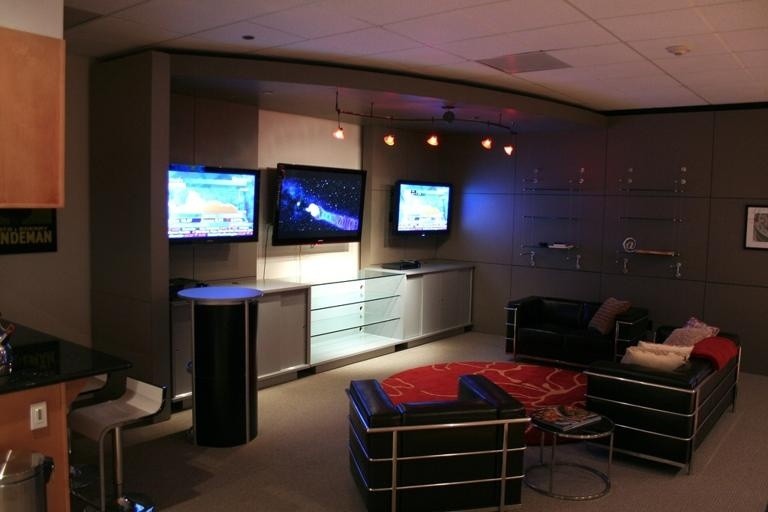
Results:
[381,361,593,445]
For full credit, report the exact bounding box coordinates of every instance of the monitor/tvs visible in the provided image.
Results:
[272,163,367,246]
[393,179,454,235]
[167,163,260,245]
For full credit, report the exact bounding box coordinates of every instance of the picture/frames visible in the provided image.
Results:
[743,203,768,250]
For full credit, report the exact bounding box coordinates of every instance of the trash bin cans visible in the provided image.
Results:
[0,450,55,512]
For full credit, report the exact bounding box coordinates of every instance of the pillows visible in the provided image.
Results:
[663,327,712,350]
[683,312,719,337]
[589,297,633,335]
[620,346,686,370]
[638,339,694,360]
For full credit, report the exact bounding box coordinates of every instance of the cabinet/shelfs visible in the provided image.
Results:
[406,266,474,347]
[522,167,584,269]
[309,275,406,374]
[617,165,687,277]
[170,287,309,409]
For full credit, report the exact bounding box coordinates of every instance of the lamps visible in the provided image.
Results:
[333,90,518,155]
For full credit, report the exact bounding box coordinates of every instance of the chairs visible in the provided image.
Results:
[67,376,167,512]
[66,373,108,455]
[344,374,532,512]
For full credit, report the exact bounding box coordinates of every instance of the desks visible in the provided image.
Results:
[0,317,132,511]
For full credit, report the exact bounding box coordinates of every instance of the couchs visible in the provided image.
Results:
[504,294,650,371]
[585,327,743,478]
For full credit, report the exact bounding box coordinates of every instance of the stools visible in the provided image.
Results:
[524,405,615,500]
